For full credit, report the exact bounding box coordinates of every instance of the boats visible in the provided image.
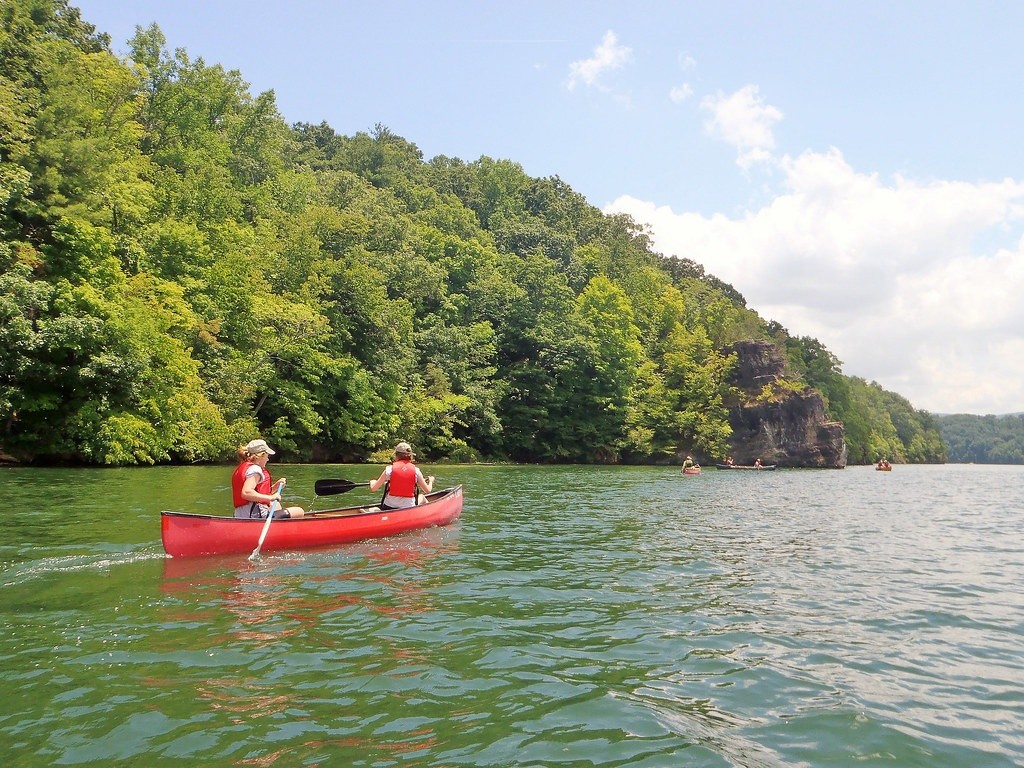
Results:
[680,468,699,474]
[160,484,463,557]
[876,466,891,471]
[716,463,777,471]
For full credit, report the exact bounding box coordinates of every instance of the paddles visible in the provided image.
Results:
[315,478,429,497]
[250,481,284,559]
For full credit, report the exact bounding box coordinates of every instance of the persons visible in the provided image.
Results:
[682,456,701,468]
[370,442,435,510]
[878,457,890,468]
[231,439,304,518]
[754,459,762,467]
[727,457,734,464]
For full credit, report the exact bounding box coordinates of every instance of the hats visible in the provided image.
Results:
[244,440,275,457]
[392,443,412,455]
